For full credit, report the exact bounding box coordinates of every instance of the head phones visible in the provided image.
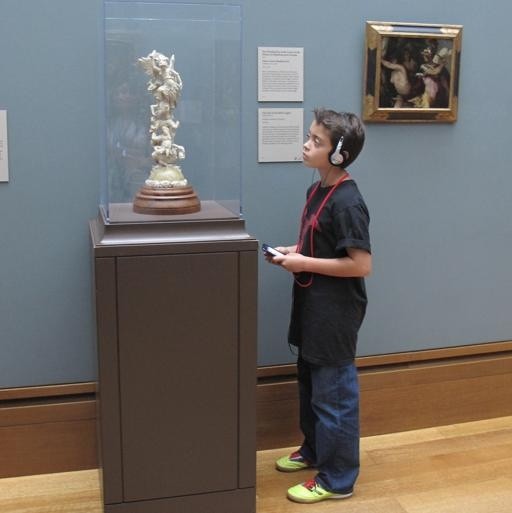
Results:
[328,135,347,166]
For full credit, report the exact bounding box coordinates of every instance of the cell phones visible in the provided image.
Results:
[261,243,284,256]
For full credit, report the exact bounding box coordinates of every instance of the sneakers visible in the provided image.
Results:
[275,450,313,472]
[286,478,353,504]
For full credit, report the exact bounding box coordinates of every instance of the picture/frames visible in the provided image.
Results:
[360,18,463,122]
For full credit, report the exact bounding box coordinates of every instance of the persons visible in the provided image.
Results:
[263,106,373,503]
[108,77,153,200]
[380,36,453,108]
[148,54,186,166]
